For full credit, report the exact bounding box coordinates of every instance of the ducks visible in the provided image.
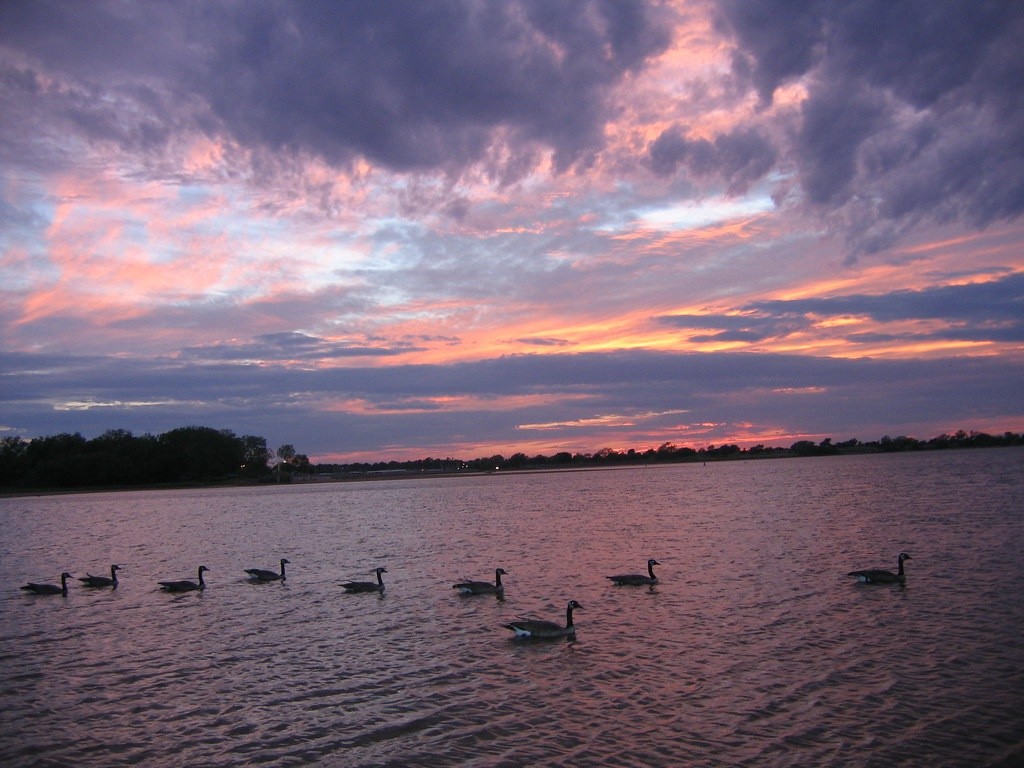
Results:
[496,600,584,641]
[19,573,74,596]
[452,568,509,596]
[158,566,210,593]
[605,560,662,585]
[336,567,389,591]
[243,559,291,583]
[76,564,122,588]
[840,552,914,586]
